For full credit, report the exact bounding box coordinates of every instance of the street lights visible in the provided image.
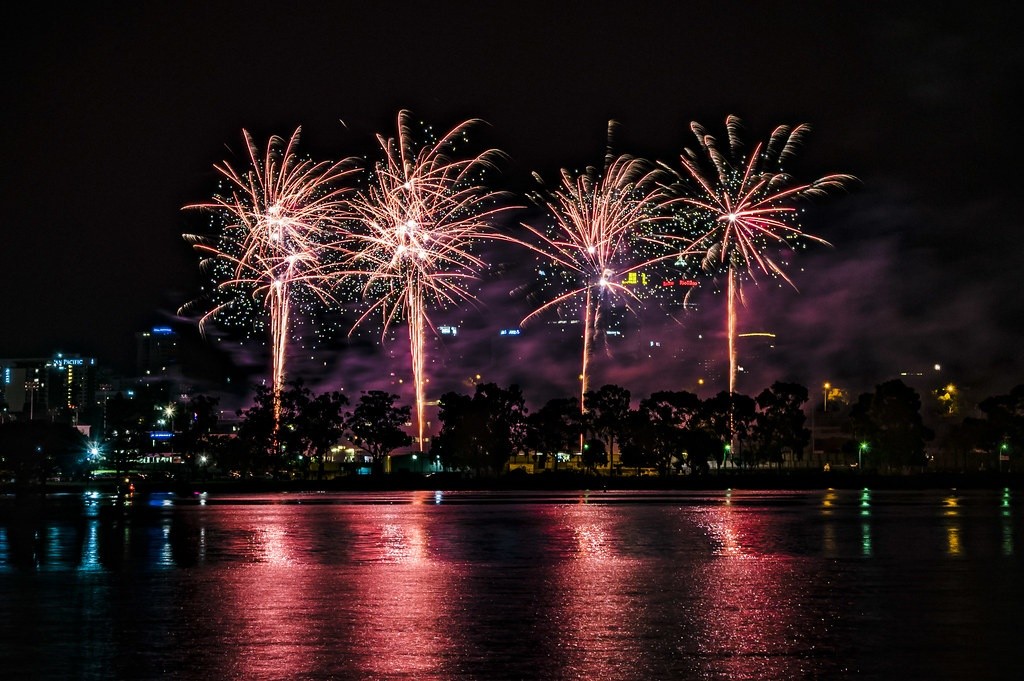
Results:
[412,454,417,472]
[947,384,954,413]
[999,445,1007,472]
[824,382,831,412]
[724,445,731,469]
[858,443,867,469]
